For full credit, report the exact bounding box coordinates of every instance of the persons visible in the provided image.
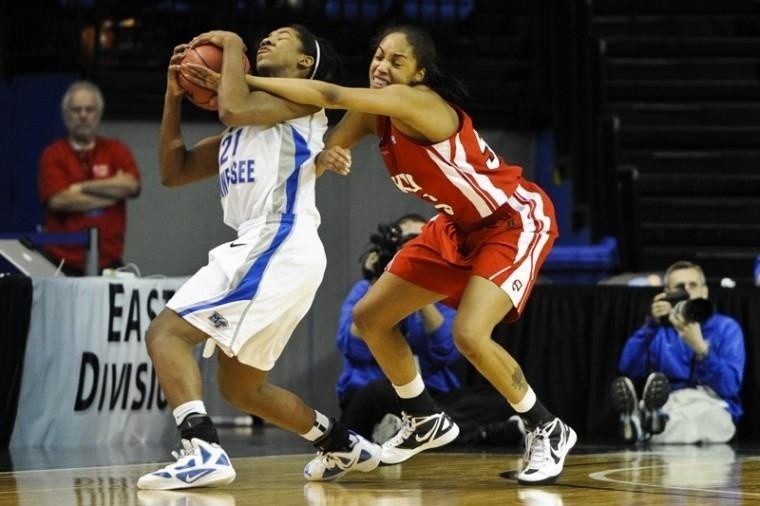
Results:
[176,24,580,488]
[609,260,750,446]
[335,212,539,454]
[135,23,386,494]
[34,76,145,276]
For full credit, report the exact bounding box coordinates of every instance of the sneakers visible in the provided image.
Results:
[136,437,236,491]
[372,411,459,466]
[493,416,526,448]
[303,430,380,482]
[612,371,670,444]
[518,418,577,483]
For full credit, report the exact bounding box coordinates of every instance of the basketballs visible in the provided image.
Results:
[177,38,251,111]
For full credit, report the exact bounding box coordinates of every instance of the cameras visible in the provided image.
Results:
[658,288,714,327]
